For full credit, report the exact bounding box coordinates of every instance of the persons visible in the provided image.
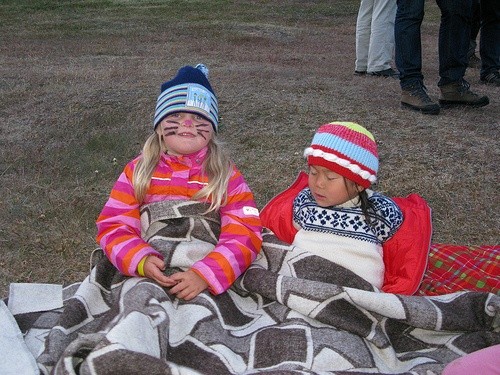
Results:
[259,121,432,296]
[355,0,500,115]
[95,63,263,300]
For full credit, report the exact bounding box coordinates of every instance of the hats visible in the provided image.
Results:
[153,63,219,131]
[303,121,379,188]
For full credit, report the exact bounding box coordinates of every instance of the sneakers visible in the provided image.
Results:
[466,56,482,68]
[354,70,366,75]
[437,77,489,108]
[480,71,500,85]
[400,78,440,114]
[367,68,399,78]
